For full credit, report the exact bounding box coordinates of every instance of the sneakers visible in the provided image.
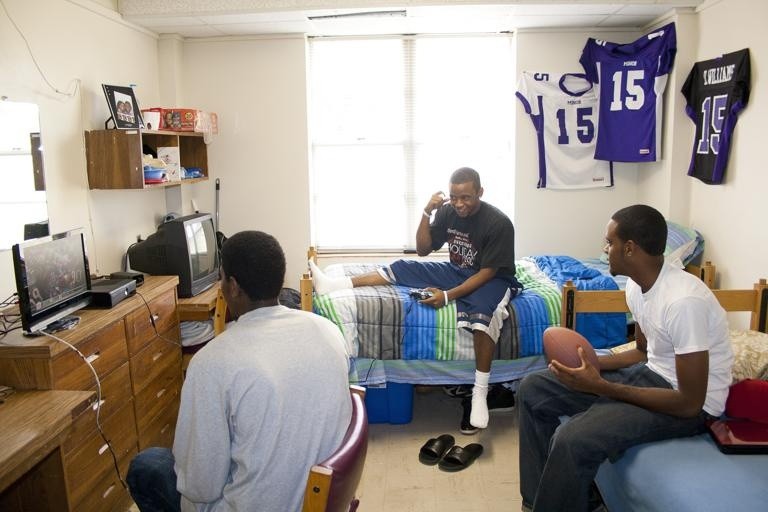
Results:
[442,384,516,434]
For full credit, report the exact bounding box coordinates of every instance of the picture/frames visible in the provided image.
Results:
[101,84,147,132]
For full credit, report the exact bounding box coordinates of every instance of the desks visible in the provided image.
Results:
[171,279,222,322]
[0,386,99,495]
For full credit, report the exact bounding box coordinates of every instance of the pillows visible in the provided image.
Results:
[663,220,706,270]
[724,330,768,385]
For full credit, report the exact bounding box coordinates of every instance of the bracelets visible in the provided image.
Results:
[443,290,448,305]
[423,210,431,218]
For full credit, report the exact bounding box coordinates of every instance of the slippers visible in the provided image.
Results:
[419,434,455,464]
[439,444,483,470]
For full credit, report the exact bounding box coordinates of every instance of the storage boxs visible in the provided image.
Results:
[164,106,218,135]
[366,379,414,425]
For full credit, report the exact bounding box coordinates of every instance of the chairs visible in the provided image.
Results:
[299,382,370,511]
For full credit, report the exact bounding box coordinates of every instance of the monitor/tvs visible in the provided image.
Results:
[128,213,219,298]
[12,226,93,336]
[24,221,48,240]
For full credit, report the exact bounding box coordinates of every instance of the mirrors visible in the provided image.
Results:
[0,96,53,256]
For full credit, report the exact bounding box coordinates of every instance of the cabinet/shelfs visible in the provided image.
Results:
[0,275,189,512]
[86,128,209,190]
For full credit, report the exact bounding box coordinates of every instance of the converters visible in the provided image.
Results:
[110,271,145,287]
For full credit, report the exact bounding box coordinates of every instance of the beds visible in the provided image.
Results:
[561,279,767,511]
[301,221,715,424]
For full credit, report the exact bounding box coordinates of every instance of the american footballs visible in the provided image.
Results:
[544,327,599,375]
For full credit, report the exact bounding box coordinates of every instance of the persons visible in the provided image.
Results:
[125,230,351,511]
[124,102,132,114]
[116,101,125,114]
[516,206,731,512]
[165,112,174,129]
[310,167,515,428]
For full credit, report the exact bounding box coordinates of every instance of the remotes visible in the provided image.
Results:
[404,250,417,253]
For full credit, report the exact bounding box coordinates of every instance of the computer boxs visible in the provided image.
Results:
[77,279,136,309]
